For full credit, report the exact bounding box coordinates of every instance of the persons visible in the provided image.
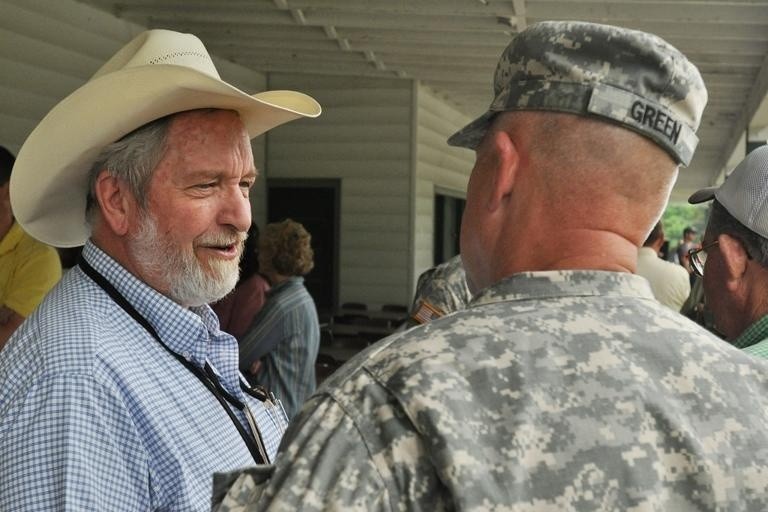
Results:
[3,20,767,511]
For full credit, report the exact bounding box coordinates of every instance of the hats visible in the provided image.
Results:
[688,143,768,241]
[447,19,709,166]
[8,29,323,250]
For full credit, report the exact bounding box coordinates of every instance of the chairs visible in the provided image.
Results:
[333,298,408,342]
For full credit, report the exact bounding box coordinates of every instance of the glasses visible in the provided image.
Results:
[685,240,723,276]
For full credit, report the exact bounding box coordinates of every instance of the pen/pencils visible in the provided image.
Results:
[269,391,277,406]
